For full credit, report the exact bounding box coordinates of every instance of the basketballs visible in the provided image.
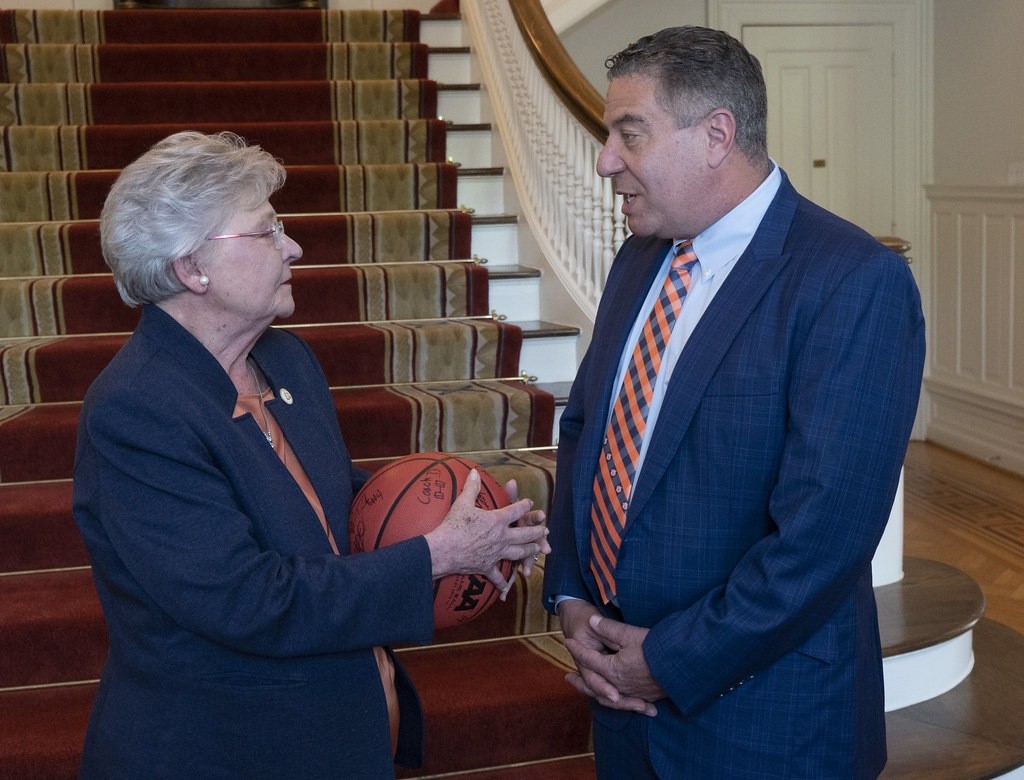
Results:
[349,451,517,630]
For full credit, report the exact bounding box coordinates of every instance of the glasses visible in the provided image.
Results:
[208,221,285,250]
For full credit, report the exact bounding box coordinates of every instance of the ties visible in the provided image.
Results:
[590,238,700,606]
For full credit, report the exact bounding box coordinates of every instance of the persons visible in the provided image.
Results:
[541,25,925,780]
[72,131,552,780]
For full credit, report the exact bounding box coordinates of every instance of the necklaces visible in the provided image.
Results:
[237,363,275,449]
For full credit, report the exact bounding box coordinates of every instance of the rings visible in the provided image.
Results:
[534,556,538,561]
